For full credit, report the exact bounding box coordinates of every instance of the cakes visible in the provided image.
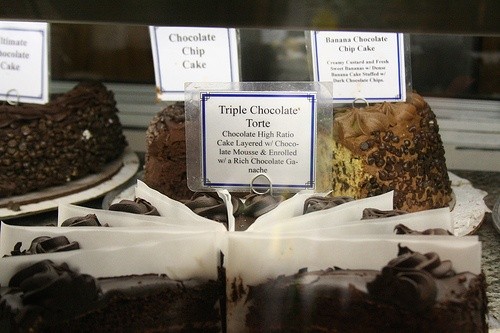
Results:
[1,79,127,205]
[318,91,457,214]
[143,99,285,201]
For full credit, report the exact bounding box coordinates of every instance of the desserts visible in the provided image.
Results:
[1,188,490,333]
